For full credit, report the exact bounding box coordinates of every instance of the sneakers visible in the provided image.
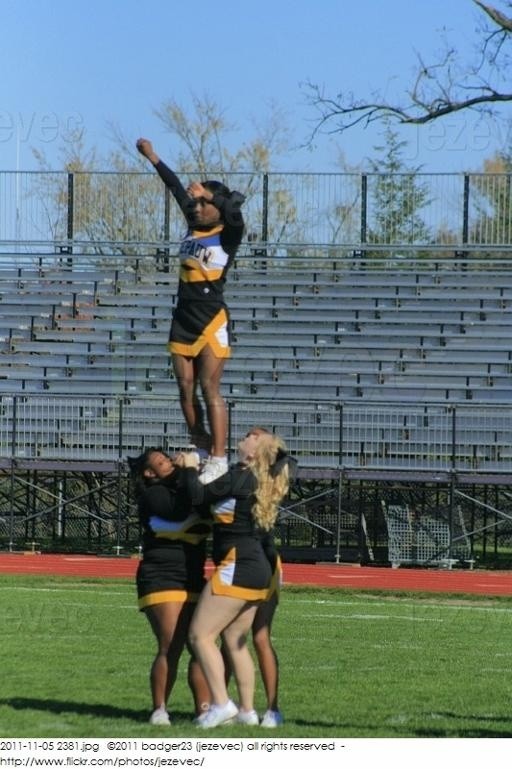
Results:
[260,710,283,728]
[149,708,170,727]
[197,455,228,484]
[191,700,239,729]
[238,708,259,726]
[190,448,207,463]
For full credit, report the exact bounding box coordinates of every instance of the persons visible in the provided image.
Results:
[126,445,212,729]
[135,136,246,487]
[218,427,297,729]
[177,428,292,731]
